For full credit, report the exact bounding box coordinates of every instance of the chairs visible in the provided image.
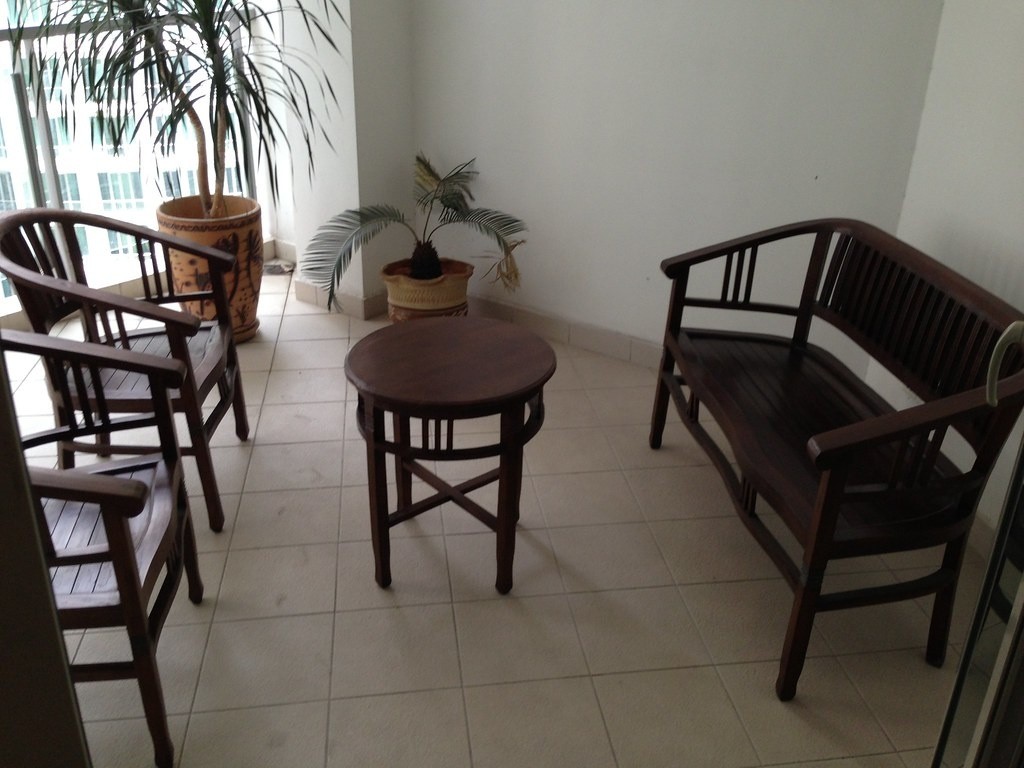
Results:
[0,206,252,533]
[0,329,204,768]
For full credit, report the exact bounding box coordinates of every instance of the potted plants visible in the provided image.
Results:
[7,0,350,343]
[298,151,528,323]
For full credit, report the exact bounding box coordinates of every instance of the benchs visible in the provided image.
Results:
[647,217,1024,701]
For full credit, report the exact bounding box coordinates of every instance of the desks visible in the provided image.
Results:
[344,313,558,595]
